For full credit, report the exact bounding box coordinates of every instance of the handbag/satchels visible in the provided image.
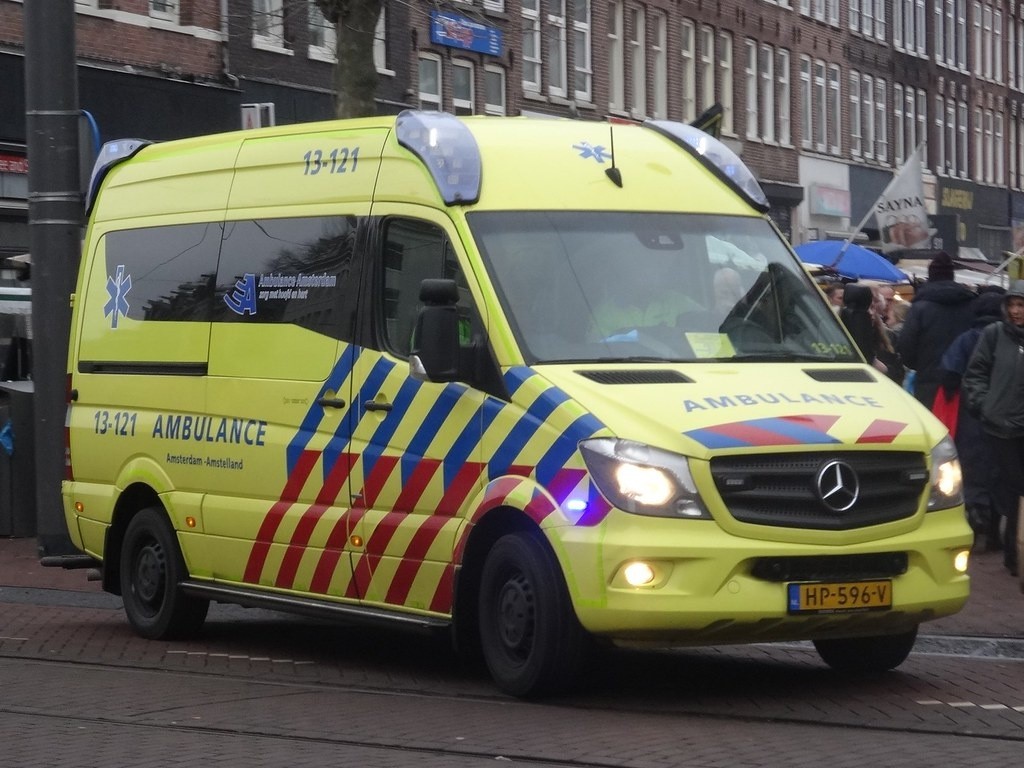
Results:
[931,385,961,440]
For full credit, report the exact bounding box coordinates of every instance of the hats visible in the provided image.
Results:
[930,251,952,282]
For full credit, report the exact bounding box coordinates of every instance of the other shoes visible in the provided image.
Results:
[984,535,1003,551]
[1005,552,1017,574]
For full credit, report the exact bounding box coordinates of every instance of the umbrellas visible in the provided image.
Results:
[796,240,912,281]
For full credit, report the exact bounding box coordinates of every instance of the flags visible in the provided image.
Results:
[873,151,931,253]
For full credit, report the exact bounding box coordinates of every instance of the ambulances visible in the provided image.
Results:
[61,110,974,698]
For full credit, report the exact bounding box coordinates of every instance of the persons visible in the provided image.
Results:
[840,249,1007,548]
[584,241,716,352]
[963,281,1023,577]
